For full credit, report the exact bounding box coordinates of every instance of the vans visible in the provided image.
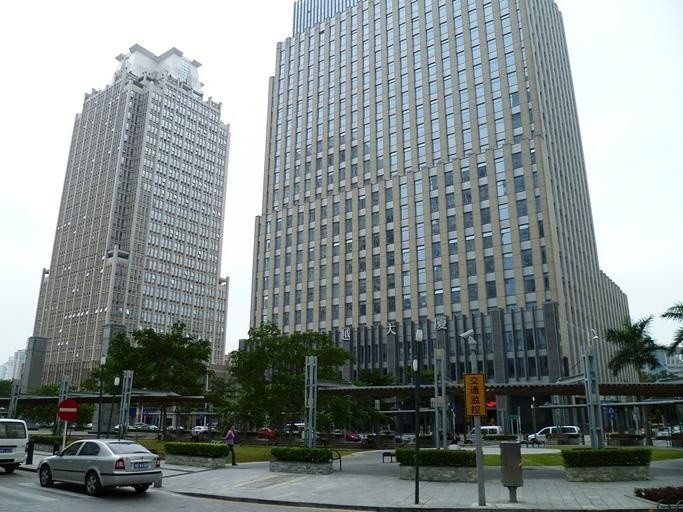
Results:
[465,426,505,444]
[526,424,585,444]
[0,418,30,473]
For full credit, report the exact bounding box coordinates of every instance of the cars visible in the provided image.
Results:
[115,420,218,435]
[37,438,163,496]
[258,422,462,443]
[641,422,683,440]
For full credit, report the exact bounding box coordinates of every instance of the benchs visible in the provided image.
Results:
[383,452,396,462]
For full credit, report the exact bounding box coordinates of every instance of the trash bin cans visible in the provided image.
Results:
[499,440,523,502]
[362,438,366,447]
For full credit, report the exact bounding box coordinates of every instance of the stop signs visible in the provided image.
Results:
[57,399,80,421]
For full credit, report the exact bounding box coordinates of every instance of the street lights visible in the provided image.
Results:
[106,374,120,438]
[410,327,424,503]
[97,355,107,440]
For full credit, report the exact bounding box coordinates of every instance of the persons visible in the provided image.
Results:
[222,423,237,466]
